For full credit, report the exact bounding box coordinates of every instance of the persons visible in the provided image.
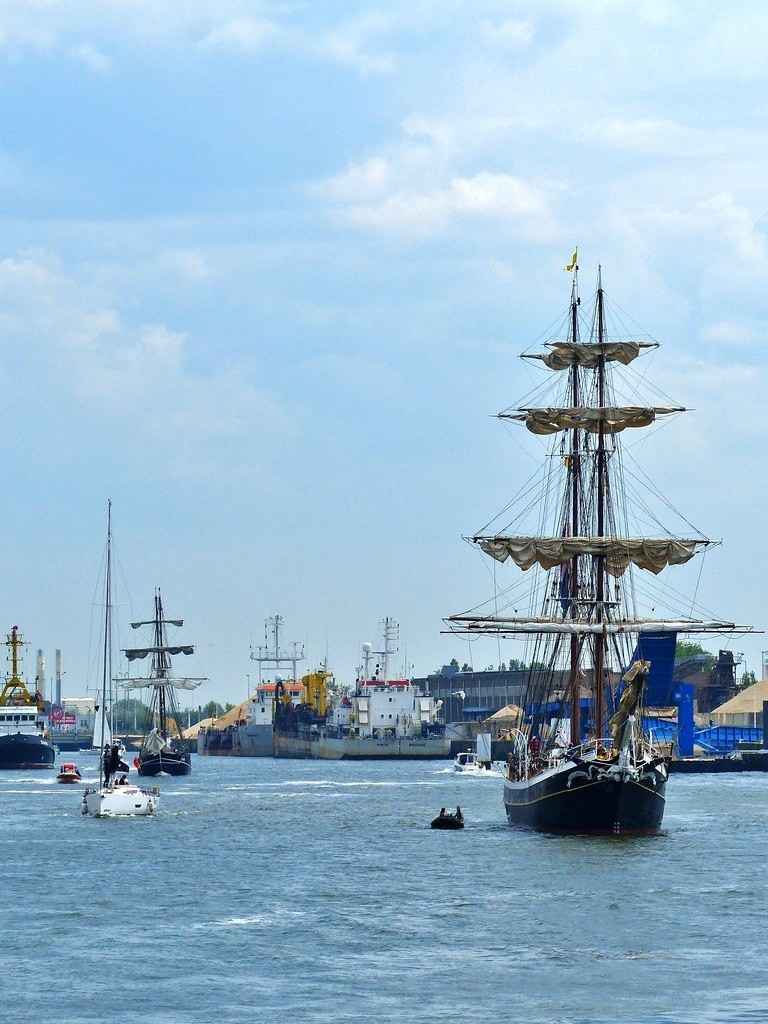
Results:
[120,775,126,785]
[529,736,540,753]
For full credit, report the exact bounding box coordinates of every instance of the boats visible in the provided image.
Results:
[56,762,82,783]
[451,748,482,774]
[431,815,465,828]
[733,739,764,751]
[195,613,453,761]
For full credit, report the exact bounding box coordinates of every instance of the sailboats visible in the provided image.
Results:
[439,249,766,833]
[80,498,166,820]
[91,690,130,773]
[112,587,210,775]
[0,623,59,772]
